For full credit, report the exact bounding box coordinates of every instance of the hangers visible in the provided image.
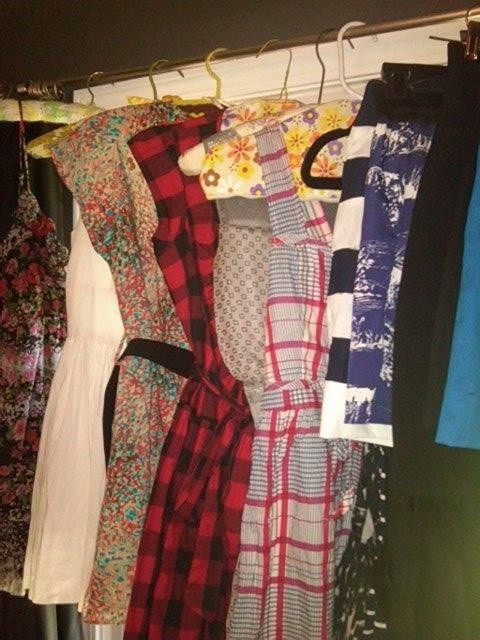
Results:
[0,17,379,190]
[430,6,480,59]
